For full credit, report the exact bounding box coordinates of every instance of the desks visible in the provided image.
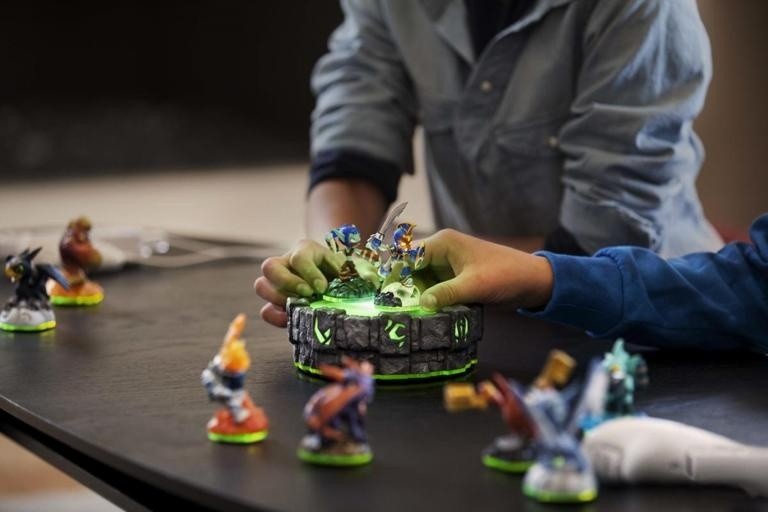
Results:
[0,221,767,510]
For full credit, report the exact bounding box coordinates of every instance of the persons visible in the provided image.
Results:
[254,0,724,329]
[407,202,768,358]
